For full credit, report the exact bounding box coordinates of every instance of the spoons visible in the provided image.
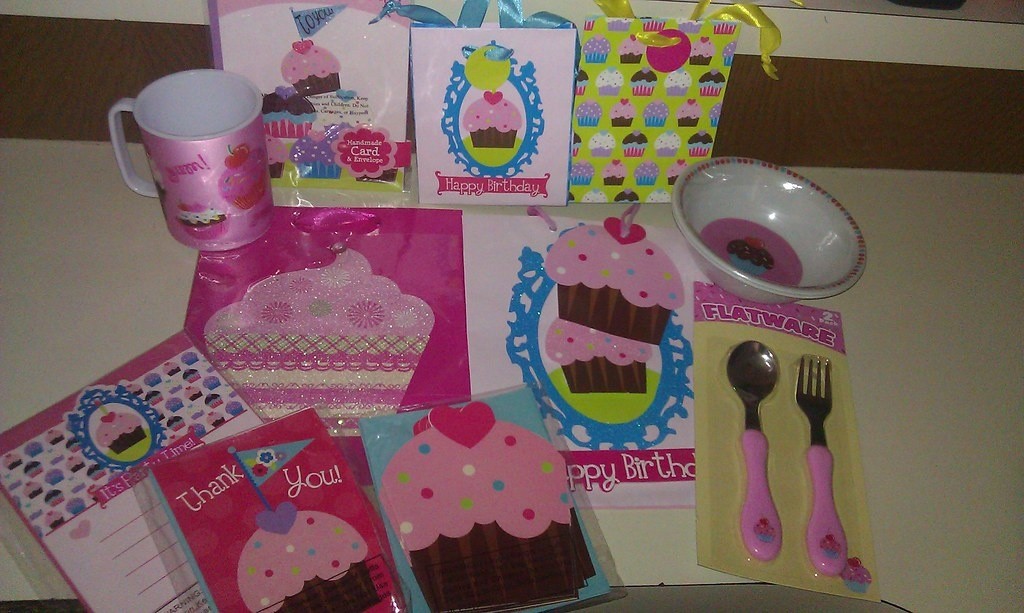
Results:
[727,340,785,561]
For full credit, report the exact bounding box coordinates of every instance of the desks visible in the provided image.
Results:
[0,13,1024,613]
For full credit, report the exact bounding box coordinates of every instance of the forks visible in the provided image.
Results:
[794,354,849,577]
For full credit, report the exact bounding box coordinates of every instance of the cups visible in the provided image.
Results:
[108,68,276,251]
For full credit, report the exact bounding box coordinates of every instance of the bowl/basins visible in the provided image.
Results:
[673,156,867,303]
